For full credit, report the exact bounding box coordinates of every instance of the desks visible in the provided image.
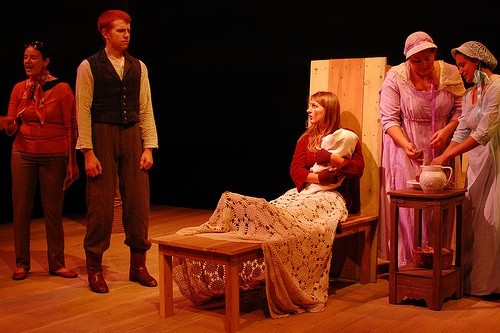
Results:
[149,214,378,333]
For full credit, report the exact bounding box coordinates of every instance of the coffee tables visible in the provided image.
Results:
[386,187,468,311]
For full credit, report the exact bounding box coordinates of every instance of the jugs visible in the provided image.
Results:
[419,165,453,193]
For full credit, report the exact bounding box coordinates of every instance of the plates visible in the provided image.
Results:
[407,179,422,189]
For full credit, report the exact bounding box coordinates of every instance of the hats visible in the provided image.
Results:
[403,31,438,59]
[451,40,497,70]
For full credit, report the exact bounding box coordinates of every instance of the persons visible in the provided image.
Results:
[378,31,467,279]
[430,40,500,299]
[218,91,365,233]
[5,38,80,280]
[74,9,159,294]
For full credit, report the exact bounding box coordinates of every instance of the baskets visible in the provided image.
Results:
[411,245,454,269]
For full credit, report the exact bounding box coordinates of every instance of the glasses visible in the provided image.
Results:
[25,40,50,57]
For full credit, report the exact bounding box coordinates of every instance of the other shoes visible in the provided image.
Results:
[49,267,78,278]
[13,267,28,279]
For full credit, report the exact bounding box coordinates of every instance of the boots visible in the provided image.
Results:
[85,252,110,292]
[128,249,158,286]
